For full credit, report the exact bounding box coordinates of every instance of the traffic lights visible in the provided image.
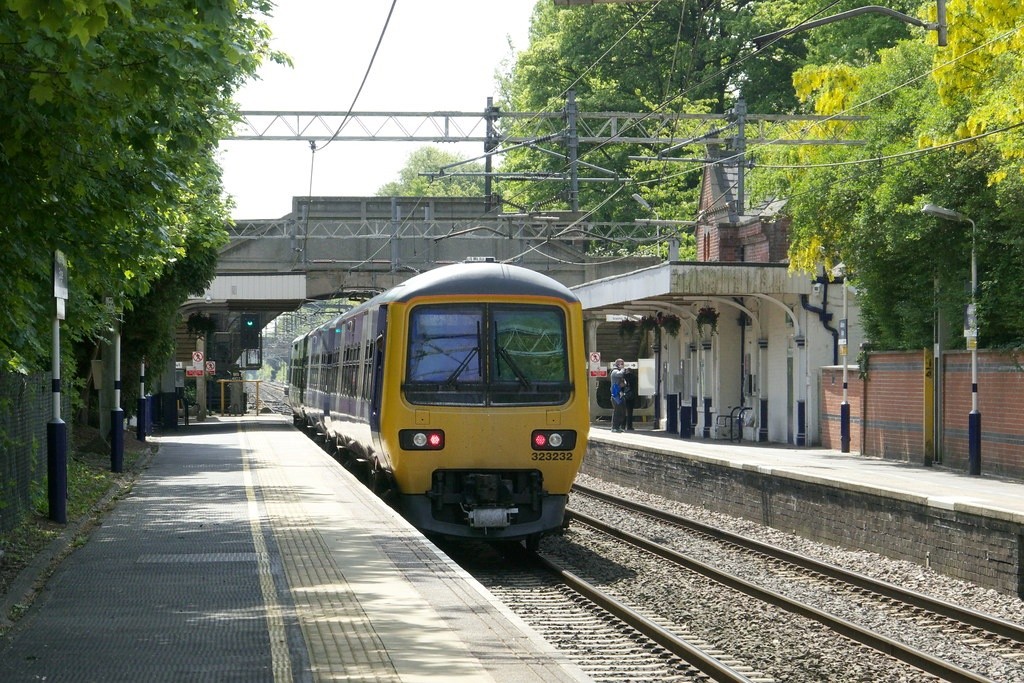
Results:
[241,312,262,348]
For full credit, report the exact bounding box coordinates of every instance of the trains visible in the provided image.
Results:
[281,257,591,553]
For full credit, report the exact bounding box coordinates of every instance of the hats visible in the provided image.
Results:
[613,359,622,365]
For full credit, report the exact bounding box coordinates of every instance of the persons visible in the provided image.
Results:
[610,359,636,432]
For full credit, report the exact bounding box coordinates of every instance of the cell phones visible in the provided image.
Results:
[615,363,619,368]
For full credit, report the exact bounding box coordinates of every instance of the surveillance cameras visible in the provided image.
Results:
[812,283,821,293]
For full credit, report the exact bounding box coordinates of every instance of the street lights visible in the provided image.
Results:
[922,202,983,476]
[831,259,852,453]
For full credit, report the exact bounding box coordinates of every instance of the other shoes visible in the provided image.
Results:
[620,426,625,432]
[628,427,634,431]
[612,428,622,433]
